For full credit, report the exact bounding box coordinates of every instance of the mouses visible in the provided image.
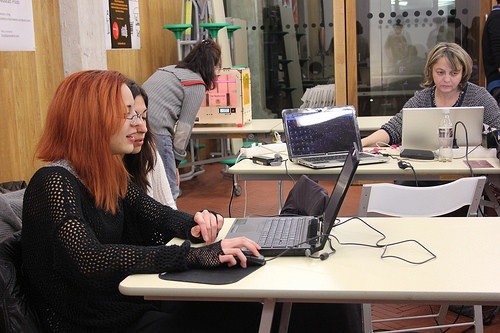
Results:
[227,252,266,266]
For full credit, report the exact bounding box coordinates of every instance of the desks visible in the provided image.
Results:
[228,146,500,216]
[176,116,398,197]
[118,217,500,333]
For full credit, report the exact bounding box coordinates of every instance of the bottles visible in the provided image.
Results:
[438,109,454,162]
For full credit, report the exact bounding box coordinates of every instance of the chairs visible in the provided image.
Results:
[279,175,329,218]
[346,176,487,333]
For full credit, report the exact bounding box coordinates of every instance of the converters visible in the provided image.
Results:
[253,155,278,166]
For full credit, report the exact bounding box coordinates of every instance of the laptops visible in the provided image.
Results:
[401,106,485,152]
[281,104,390,169]
[222,141,360,258]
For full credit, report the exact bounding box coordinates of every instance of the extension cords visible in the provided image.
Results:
[437,146,497,160]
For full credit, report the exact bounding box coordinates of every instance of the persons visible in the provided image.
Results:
[357,15,473,76]
[361,42,500,217]
[140,39,222,204]
[23,69,262,333]
[481,0,500,108]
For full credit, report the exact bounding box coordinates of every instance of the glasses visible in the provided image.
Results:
[116,113,138,122]
